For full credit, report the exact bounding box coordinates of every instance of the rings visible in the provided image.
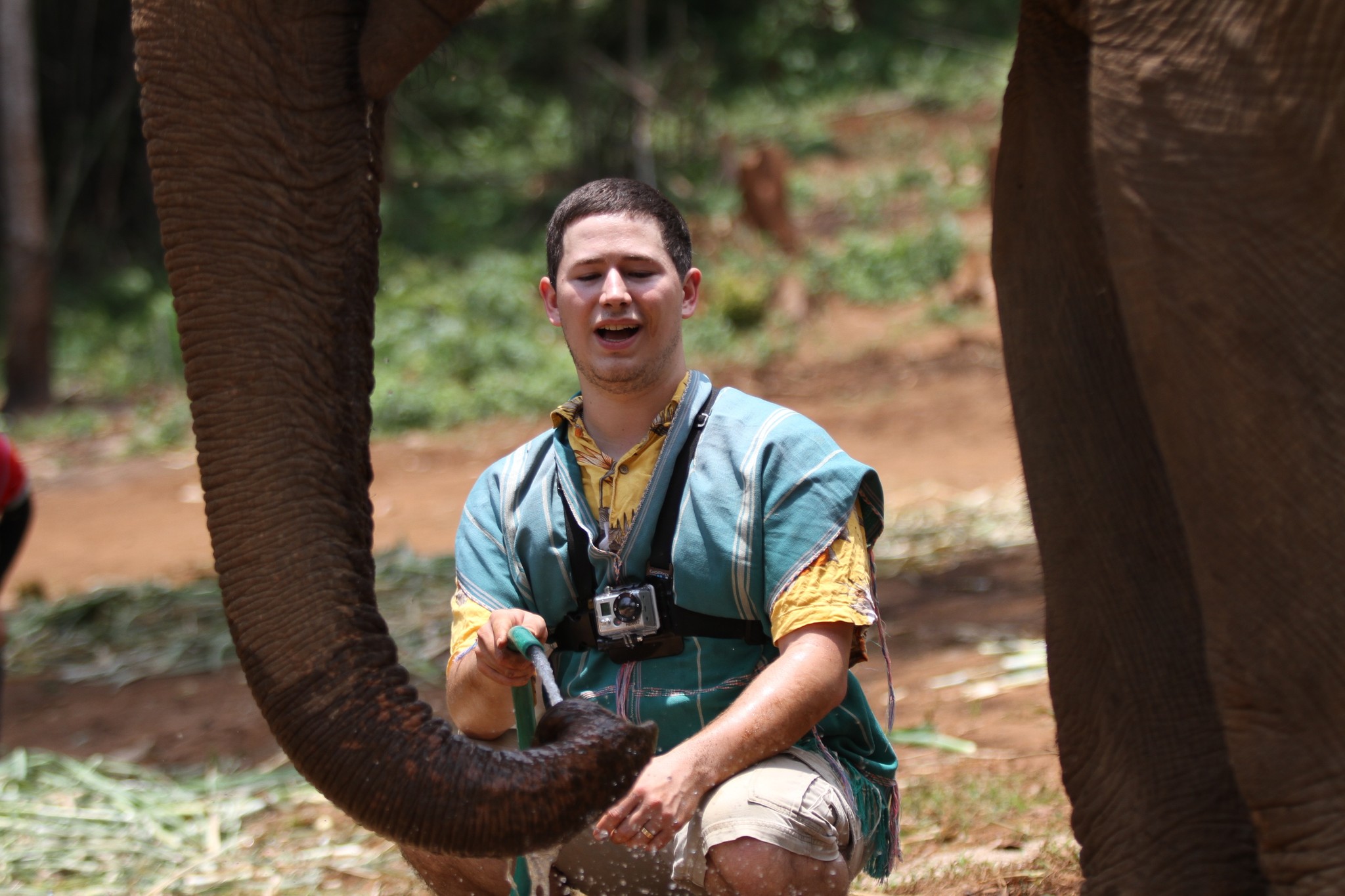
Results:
[641,826,654,839]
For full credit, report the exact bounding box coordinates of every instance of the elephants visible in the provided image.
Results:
[122,0,1345,896]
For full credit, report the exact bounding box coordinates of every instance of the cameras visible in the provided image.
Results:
[594,584,661,639]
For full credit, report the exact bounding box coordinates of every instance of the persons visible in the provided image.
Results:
[0,431,34,600]
[394,177,900,896]
